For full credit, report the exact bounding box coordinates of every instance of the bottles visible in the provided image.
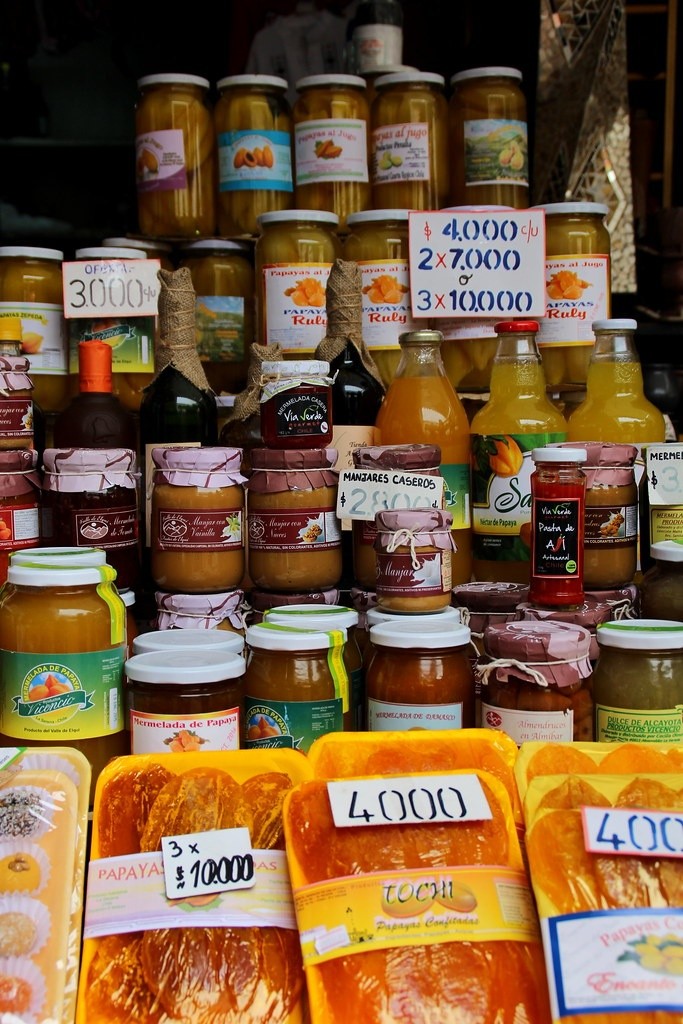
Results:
[44,340,136,589]
[135,266,220,617]
[370,329,472,587]
[221,344,287,475]
[346,0,405,78]
[313,259,386,585]
[471,320,568,587]
[0,316,35,446]
[568,318,666,442]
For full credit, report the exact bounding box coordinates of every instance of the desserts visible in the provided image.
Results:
[0,786,55,1022]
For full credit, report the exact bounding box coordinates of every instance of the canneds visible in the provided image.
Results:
[0,546,137,810]
[0,247,156,586]
[148,360,683,619]
[124,581,683,755]
[138,1,529,240]
[182,202,613,391]
